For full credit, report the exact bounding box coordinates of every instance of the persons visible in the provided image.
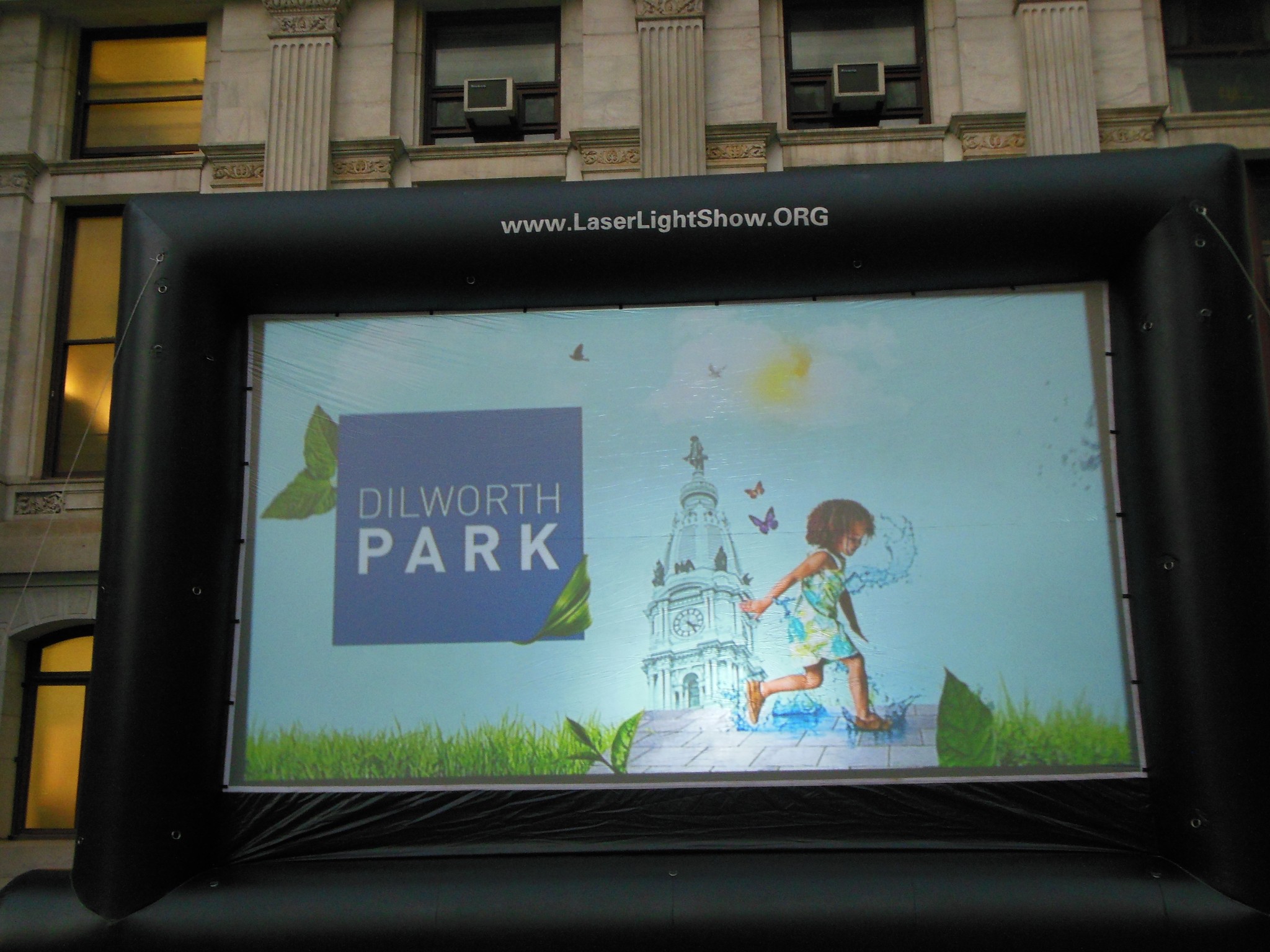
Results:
[735,496,894,731]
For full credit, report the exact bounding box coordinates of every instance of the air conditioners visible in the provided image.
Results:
[829,61,885,111]
[464,77,521,127]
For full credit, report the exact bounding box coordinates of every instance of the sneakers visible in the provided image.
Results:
[855,714,894,731]
[746,679,764,722]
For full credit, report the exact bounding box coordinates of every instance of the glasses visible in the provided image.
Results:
[843,534,863,545]
